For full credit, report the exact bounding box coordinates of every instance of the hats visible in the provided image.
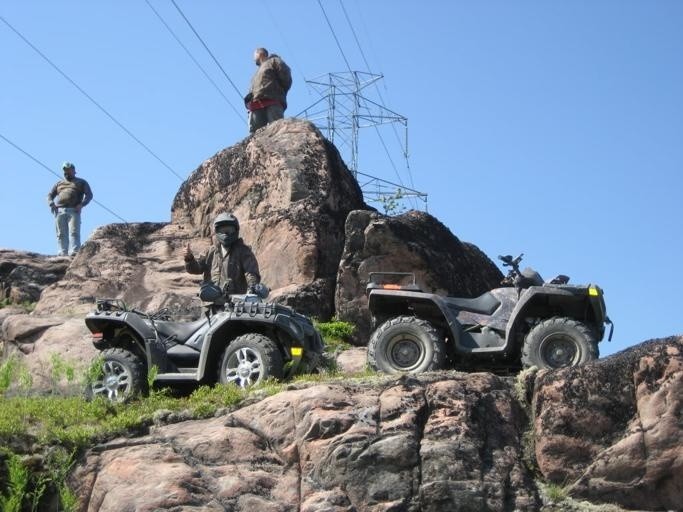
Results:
[62,162,75,171]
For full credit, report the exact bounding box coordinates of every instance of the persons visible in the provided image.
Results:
[243,48,293,135]
[45,161,93,259]
[181,211,270,317]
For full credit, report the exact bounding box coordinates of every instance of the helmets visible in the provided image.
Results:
[213,211,241,246]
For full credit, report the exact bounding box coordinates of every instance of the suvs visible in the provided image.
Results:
[365,254,614,373]
[83,283,325,406]
